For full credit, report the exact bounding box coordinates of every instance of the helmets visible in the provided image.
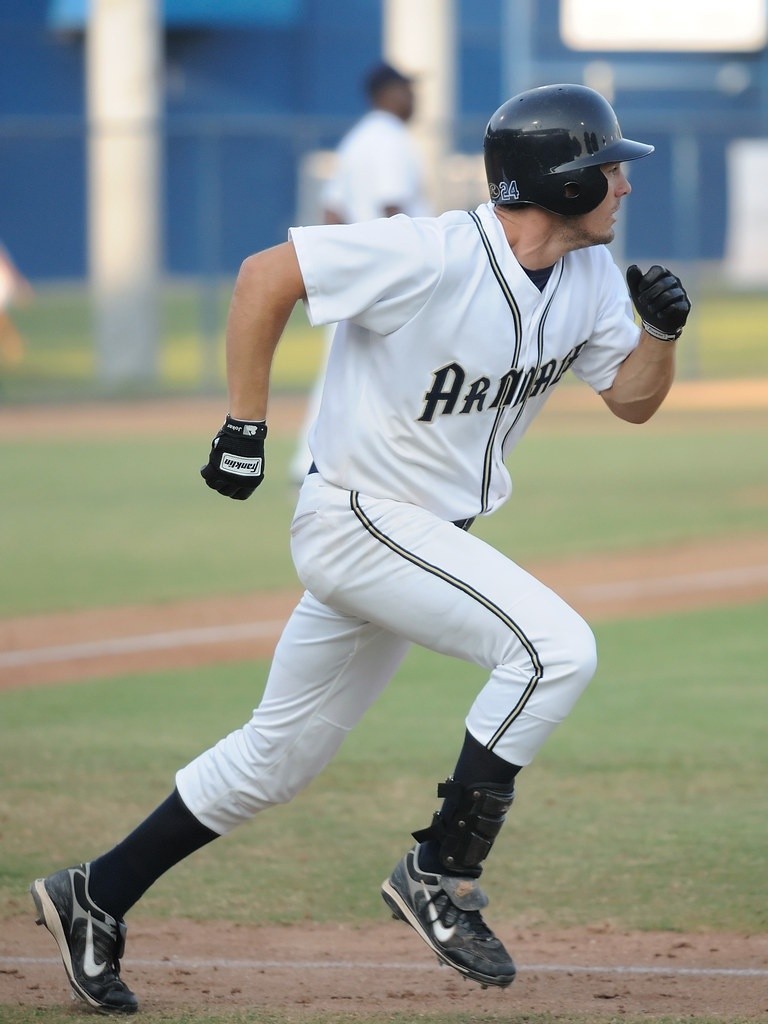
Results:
[485,85,656,215]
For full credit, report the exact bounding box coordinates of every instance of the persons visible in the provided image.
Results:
[31,83,689,1014]
[292,63,430,486]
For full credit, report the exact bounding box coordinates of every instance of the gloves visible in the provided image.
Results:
[201,414,266,501]
[626,264,690,341]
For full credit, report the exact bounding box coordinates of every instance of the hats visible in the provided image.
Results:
[370,64,407,95]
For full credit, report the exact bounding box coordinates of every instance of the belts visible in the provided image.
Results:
[307,460,478,532]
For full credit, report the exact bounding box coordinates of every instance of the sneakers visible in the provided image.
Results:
[382,845,515,988]
[30,864,139,1017]
